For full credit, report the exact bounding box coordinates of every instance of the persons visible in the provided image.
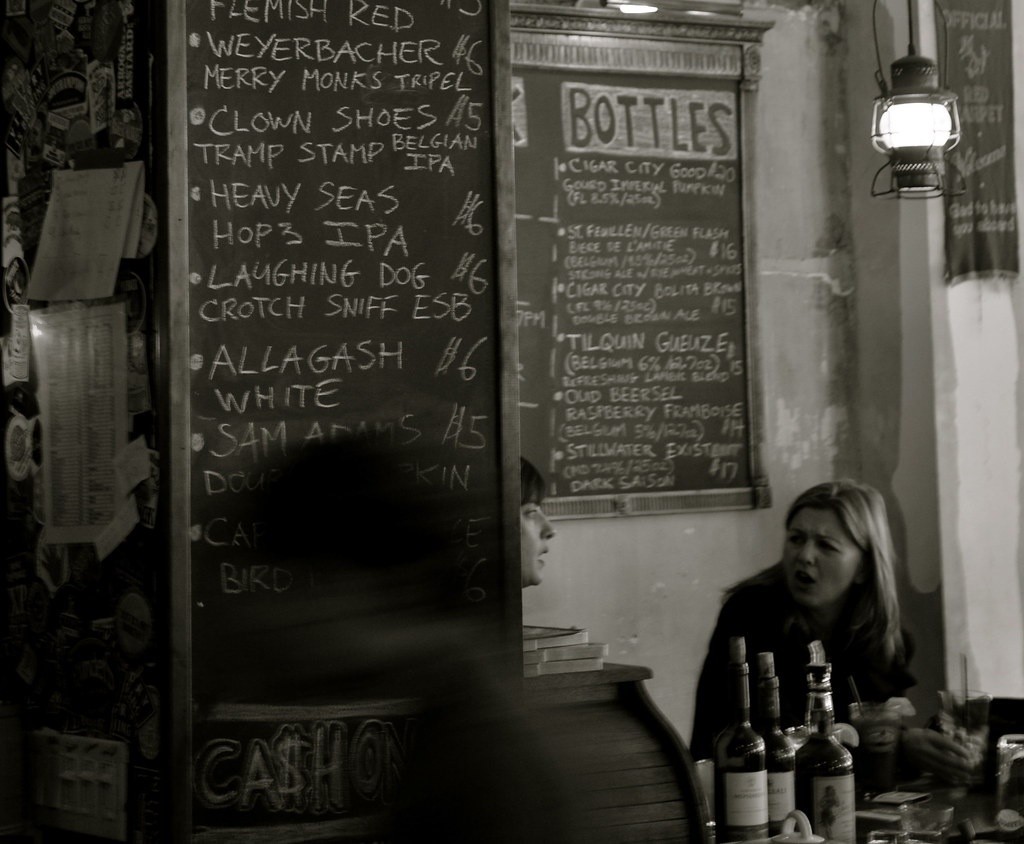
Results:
[519,454,558,589]
[690,478,976,801]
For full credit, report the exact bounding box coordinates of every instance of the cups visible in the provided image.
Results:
[898,802,954,843]
[938,689,993,789]
[847,702,899,796]
[996,735,1024,839]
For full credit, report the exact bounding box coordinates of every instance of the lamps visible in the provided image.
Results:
[870,56,962,201]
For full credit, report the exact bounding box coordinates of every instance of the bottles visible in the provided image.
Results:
[751,652,797,840]
[796,640,855,844]
[714,637,768,843]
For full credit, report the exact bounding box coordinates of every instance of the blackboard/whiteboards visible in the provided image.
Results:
[158,2,528,844]
[501,4,777,520]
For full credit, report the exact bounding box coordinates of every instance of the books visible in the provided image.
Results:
[522,622,609,677]
